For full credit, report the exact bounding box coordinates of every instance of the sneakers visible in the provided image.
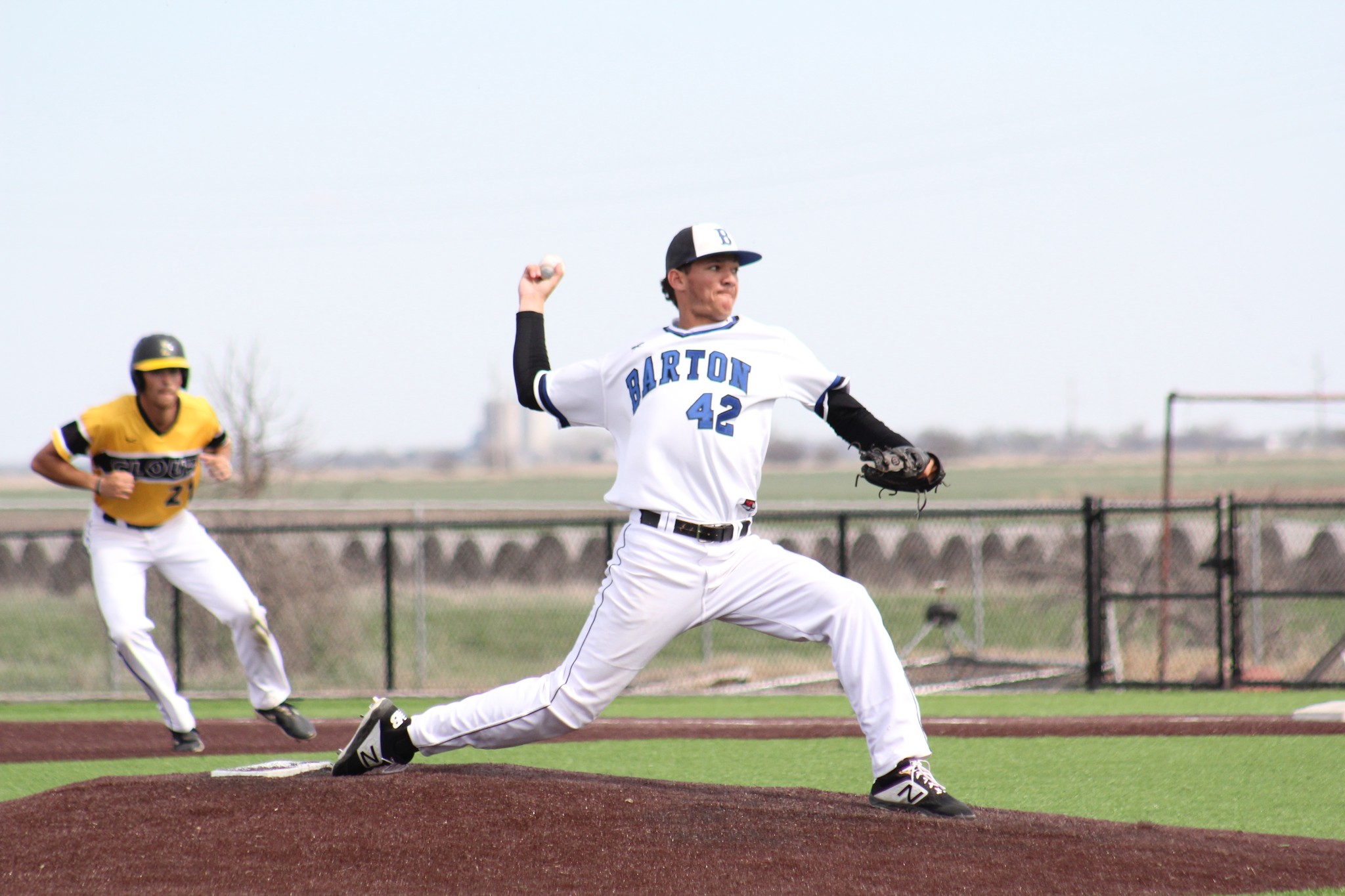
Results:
[869,758,975,820]
[170,728,204,753]
[255,702,317,741]
[331,695,414,776]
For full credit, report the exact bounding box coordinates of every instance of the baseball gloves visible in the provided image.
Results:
[848,441,951,520]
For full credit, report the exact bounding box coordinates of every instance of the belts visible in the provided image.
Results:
[639,508,750,542]
[104,514,154,529]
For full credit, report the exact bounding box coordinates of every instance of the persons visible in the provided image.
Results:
[30,335,317,752]
[331,224,975,819]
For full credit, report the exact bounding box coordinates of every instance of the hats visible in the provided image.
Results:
[666,225,762,274]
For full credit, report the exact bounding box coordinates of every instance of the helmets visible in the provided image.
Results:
[131,335,189,392]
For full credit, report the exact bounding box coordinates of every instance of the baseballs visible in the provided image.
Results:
[538,253,567,281]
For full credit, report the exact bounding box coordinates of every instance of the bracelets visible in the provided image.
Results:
[96,476,102,496]
